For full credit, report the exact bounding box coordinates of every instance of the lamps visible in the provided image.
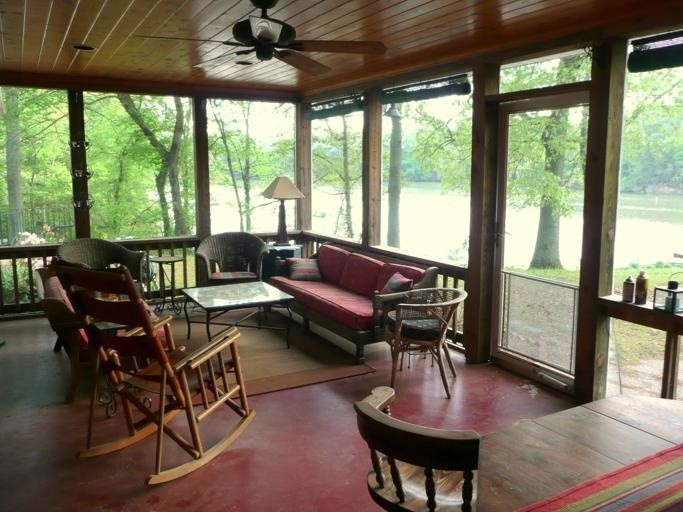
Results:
[261,174,307,246]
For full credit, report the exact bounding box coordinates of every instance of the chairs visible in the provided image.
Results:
[384,286,471,399]
[350,382,480,512]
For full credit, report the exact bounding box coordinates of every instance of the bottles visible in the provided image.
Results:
[635,270,649,305]
[621,275,635,303]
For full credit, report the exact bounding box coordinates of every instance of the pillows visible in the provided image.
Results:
[285,256,323,282]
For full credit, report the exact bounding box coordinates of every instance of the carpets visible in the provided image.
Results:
[75,302,376,410]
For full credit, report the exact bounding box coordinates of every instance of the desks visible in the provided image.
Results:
[479,396,683,512]
[262,242,305,274]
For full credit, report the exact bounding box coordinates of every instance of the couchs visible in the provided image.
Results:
[265,241,441,363]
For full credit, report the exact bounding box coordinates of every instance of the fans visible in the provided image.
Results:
[130,1,392,82]
